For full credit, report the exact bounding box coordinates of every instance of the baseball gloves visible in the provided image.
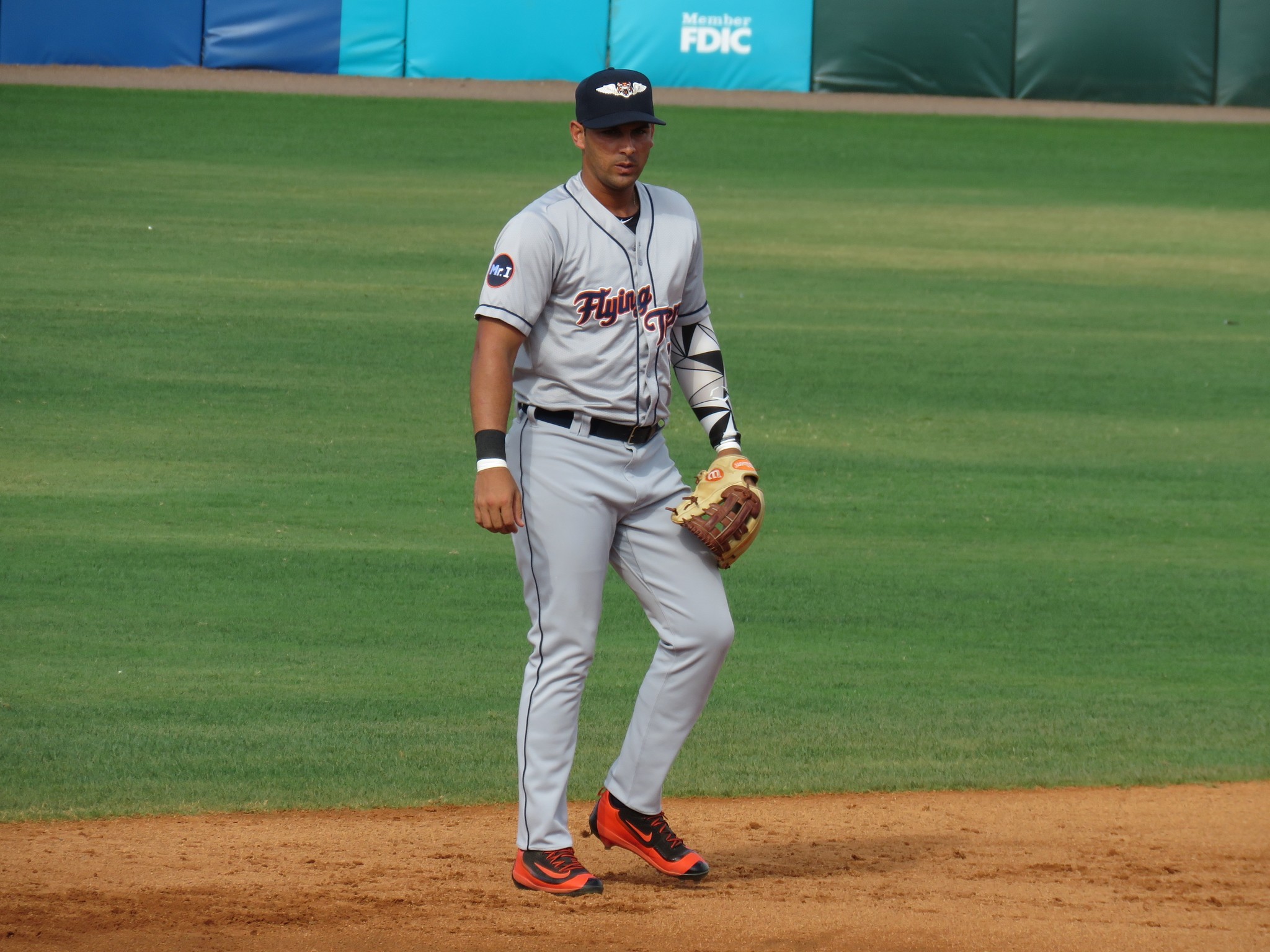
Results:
[670,454,766,570]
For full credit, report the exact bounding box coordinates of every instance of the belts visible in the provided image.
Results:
[517,400,663,446]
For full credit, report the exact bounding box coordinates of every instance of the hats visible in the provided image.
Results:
[574,65,669,130]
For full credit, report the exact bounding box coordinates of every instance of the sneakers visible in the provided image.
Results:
[510,840,604,897]
[588,783,710,882]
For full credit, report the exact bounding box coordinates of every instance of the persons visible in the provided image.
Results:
[468,66,767,896]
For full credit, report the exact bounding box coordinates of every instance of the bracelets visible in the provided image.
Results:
[476,458,508,473]
[474,429,509,463]
